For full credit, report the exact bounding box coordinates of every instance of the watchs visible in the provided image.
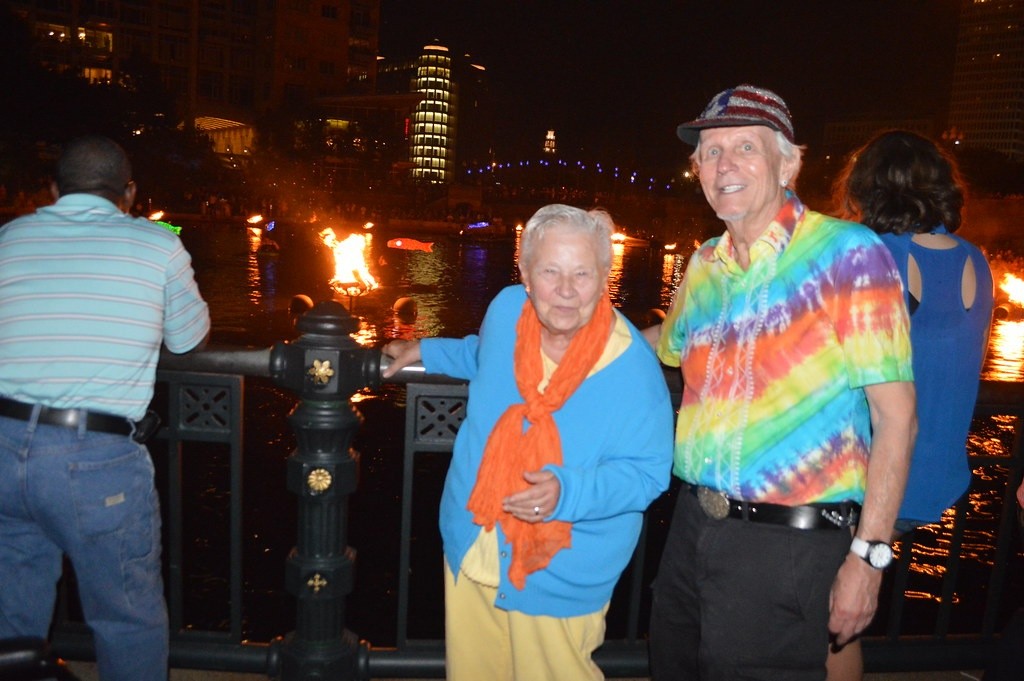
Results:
[851,536,894,571]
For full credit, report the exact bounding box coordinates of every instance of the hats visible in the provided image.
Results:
[677,83,795,146]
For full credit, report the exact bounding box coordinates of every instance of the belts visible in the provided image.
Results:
[689,484,857,530]
[0,397,140,436]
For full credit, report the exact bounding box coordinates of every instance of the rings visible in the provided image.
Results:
[535,507,539,515]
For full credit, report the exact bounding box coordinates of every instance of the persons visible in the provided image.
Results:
[134,187,481,224]
[380,203,675,681]
[0,173,61,211]
[833,127,993,544]
[637,83,919,681]
[0,134,213,681]
